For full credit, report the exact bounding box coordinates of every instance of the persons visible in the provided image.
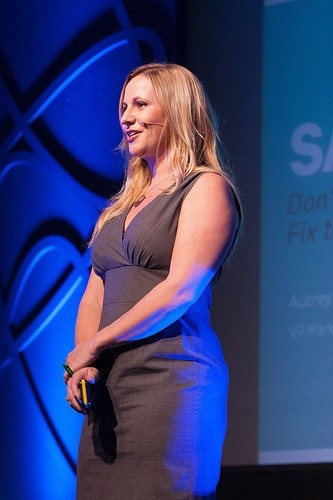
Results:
[63,64,242,500]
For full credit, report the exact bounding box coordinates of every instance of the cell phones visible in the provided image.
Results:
[81,379,91,408]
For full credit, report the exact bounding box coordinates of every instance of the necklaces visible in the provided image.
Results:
[133,174,172,207]
